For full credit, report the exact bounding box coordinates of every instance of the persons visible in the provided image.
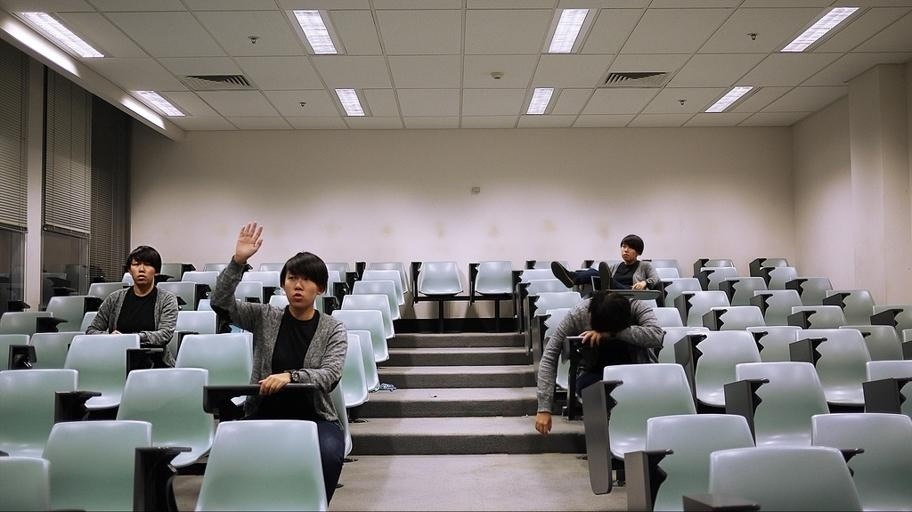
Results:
[85,245,179,369]
[534,291,665,438]
[209,220,348,507]
[551,234,661,291]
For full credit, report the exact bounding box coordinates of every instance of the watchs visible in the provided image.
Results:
[284,368,301,383]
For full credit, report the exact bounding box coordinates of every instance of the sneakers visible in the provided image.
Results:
[551,261,574,289]
[599,262,610,292]
